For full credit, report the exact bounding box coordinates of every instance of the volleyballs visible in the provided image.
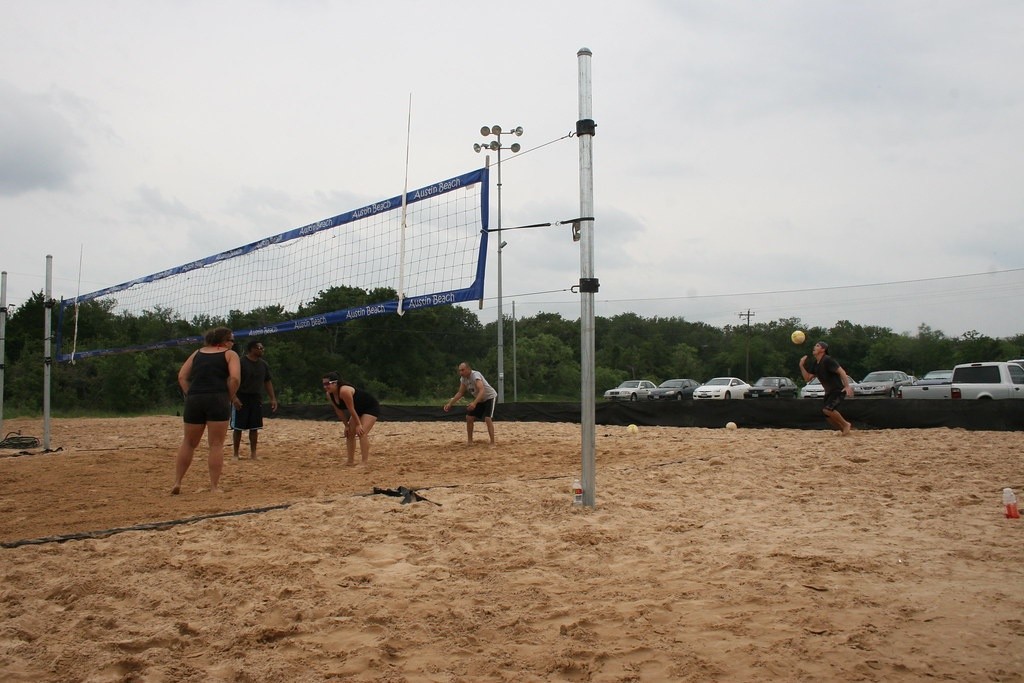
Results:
[790,330,806,345]
[726,421,737,430]
[626,423,639,434]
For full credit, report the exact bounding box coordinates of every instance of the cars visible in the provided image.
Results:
[855,370,919,399]
[749,377,799,399]
[647,378,702,401]
[604,379,658,402]
[693,377,753,400]
[800,374,858,398]
[917,370,954,385]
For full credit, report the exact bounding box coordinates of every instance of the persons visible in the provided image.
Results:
[322,370,381,464]
[443,363,498,449]
[230,340,277,461]
[799,340,856,435]
[170,326,240,493]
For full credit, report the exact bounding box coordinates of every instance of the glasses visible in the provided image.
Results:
[228,339,234,343]
[323,379,338,387]
[253,347,264,350]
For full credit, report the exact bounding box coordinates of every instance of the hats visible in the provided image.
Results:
[818,341,829,356]
